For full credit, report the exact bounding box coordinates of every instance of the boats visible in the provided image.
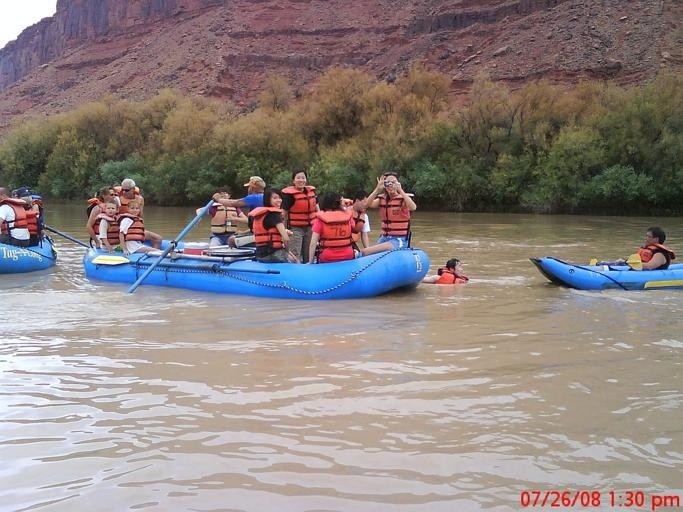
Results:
[528,255,682,292]
[0,229,57,274]
[81,239,430,301]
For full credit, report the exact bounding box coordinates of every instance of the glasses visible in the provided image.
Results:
[106,193,117,197]
[645,234,655,238]
[384,172,398,177]
[121,187,133,193]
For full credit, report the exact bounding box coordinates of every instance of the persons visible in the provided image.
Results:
[362,172,417,256]
[195,168,371,264]
[0,186,44,248]
[422,258,469,284]
[95,199,119,253]
[638,227,671,270]
[118,179,144,218]
[86,185,117,249]
[118,199,159,257]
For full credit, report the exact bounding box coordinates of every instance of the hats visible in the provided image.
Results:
[15,186,31,197]
[243,176,265,190]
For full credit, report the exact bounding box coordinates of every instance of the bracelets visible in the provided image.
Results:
[369,192,378,200]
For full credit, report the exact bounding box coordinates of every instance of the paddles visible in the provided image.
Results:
[91,253,281,276]
[589,254,642,271]
